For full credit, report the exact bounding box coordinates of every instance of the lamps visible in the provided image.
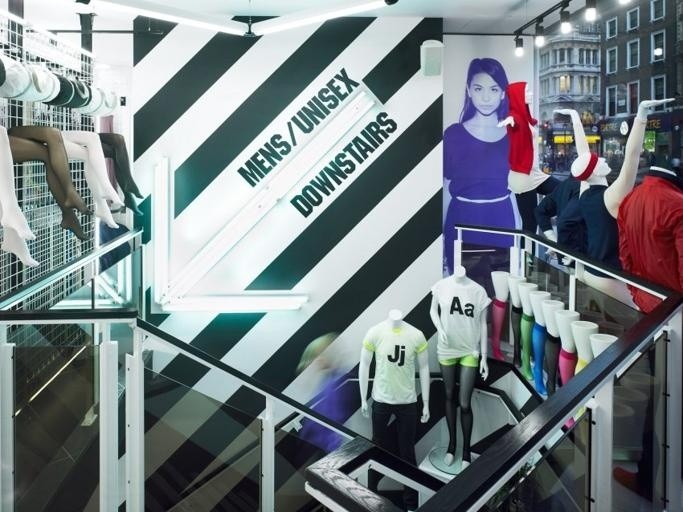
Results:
[513,0,631,59]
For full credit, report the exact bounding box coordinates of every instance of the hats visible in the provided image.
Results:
[0,53,117,116]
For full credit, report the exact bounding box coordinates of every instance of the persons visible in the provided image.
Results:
[7,126,93,240]
[359,309,430,512]
[98,132,145,217]
[429,266,494,472]
[0,125,40,267]
[534,172,581,244]
[441,57,522,273]
[616,161,683,489]
[490,270,620,400]
[59,131,125,229]
[551,98,675,271]
